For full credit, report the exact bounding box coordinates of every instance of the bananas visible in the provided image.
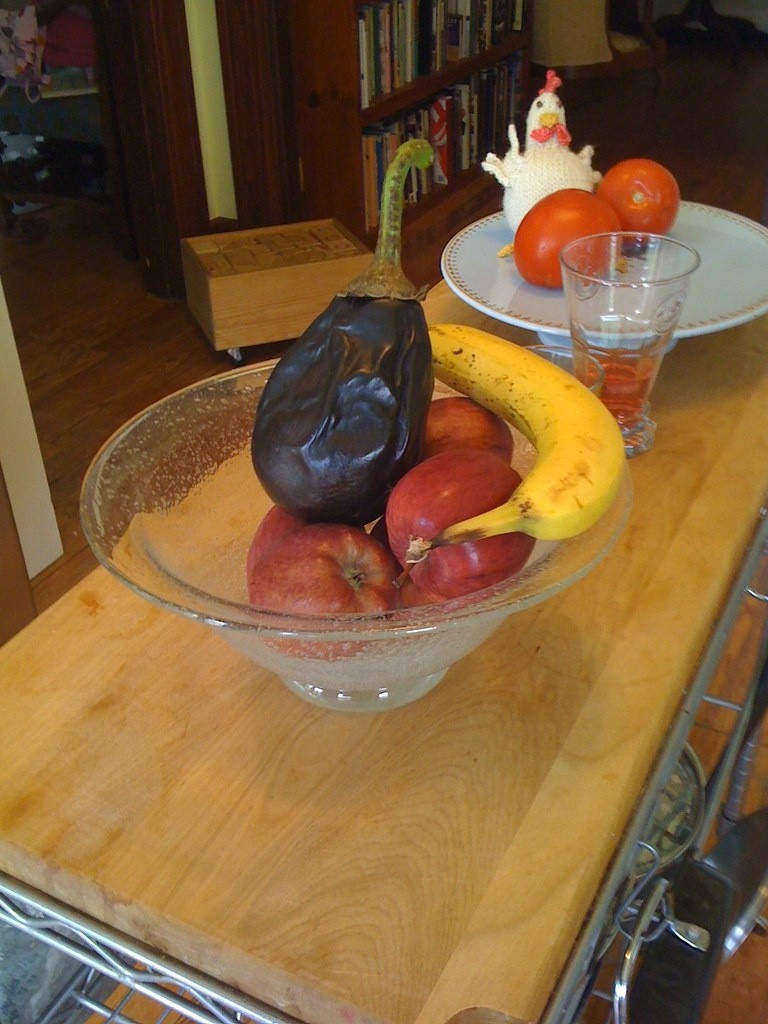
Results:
[405,320,626,568]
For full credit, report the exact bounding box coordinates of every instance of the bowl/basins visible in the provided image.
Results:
[77,356,634,710]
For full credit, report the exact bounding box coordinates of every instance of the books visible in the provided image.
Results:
[354,0,523,233]
[37,80,102,98]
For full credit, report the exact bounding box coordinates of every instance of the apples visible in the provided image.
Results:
[246,396,537,659]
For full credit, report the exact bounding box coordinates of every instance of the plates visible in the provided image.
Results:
[441,202,768,354]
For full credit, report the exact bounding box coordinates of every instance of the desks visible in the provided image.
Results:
[0,280,768,1023]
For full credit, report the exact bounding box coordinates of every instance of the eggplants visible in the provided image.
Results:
[252,139,436,528]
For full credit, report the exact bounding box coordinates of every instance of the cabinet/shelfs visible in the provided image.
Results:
[289,1,531,247]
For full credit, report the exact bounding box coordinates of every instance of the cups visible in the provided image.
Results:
[559,231,700,457]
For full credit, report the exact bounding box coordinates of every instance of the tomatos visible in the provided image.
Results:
[514,188,622,289]
[598,157,679,244]
